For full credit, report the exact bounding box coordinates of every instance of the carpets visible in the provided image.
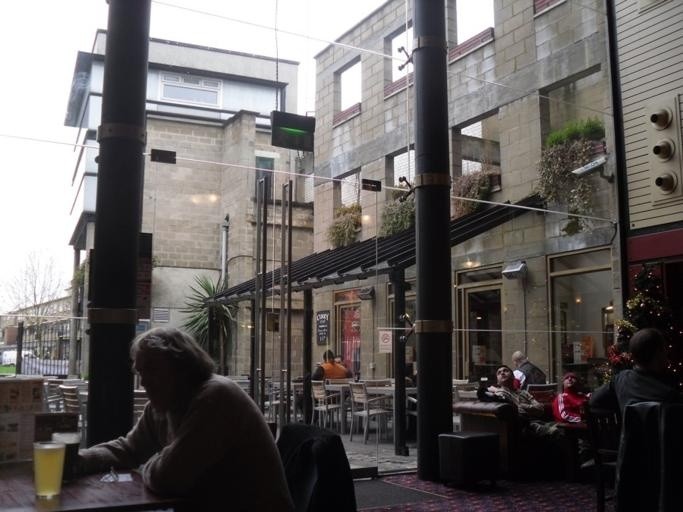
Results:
[357,472,617,512]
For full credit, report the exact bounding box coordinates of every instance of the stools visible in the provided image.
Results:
[439,431,499,489]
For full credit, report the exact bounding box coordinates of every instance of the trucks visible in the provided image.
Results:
[2,350,36,367]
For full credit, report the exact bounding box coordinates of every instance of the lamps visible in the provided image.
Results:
[502,261,527,280]
[357,286,375,300]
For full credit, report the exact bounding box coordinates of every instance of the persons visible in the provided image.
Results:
[334,355,347,367]
[77,324,296,512]
[588,327,683,451]
[552,370,591,429]
[477,366,567,440]
[511,350,546,391]
[313,349,354,379]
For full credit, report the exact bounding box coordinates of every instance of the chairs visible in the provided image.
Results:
[278,424,334,512]
[592,401,683,512]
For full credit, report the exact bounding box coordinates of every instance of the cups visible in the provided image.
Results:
[32,440,67,499]
[50,431,82,483]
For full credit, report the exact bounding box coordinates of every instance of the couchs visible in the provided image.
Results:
[454,400,555,480]
